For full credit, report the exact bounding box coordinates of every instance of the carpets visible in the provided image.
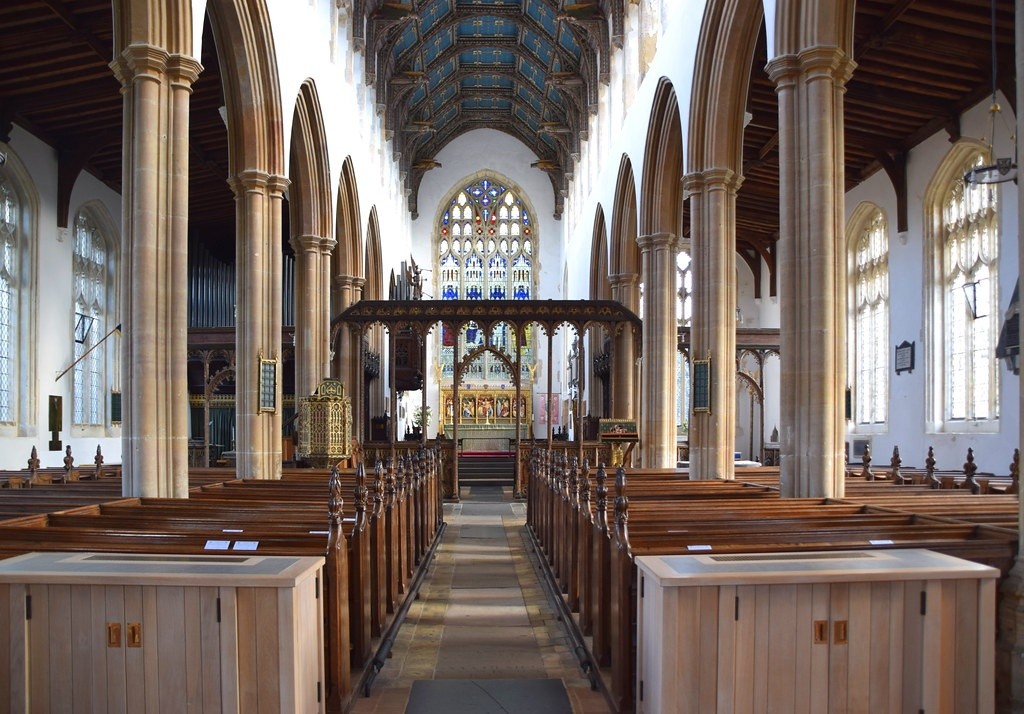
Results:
[458,450,516,458]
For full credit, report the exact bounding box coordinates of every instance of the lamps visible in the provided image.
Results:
[962,0,1018,190]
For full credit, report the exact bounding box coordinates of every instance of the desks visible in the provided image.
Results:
[0,552,327,714]
[444,424,528,450]
[632,550,1000,714]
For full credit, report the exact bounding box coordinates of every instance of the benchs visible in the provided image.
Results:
[0,438,1024,714]
